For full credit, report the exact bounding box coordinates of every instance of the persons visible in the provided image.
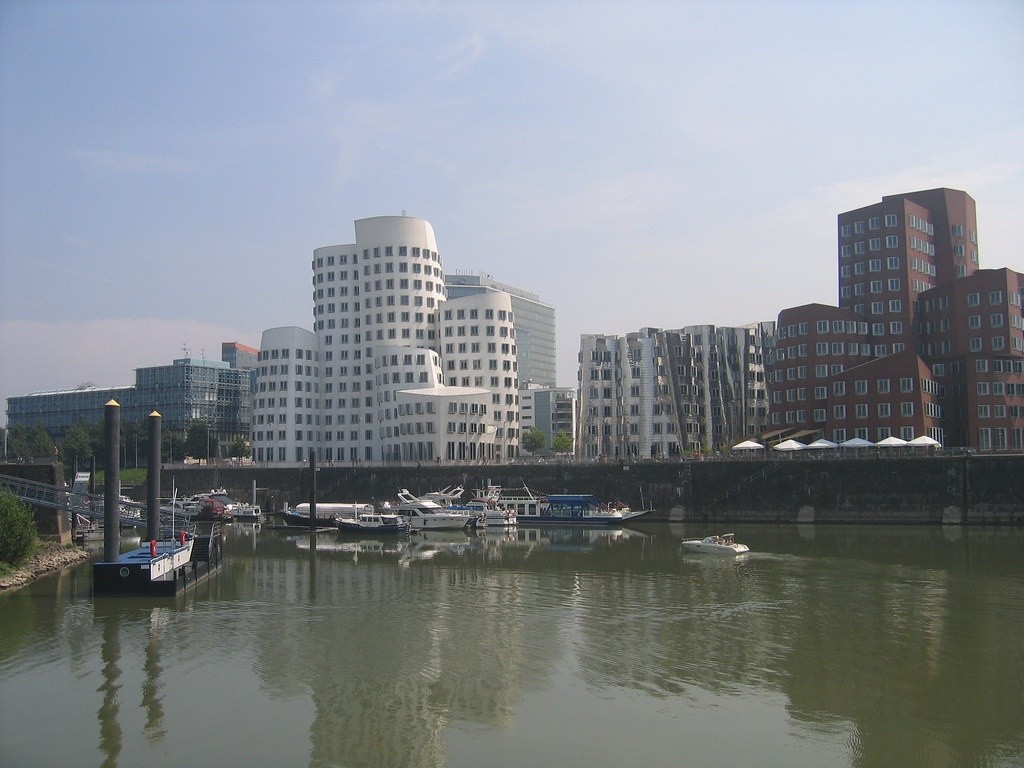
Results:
[693,451,701,462]
[716,450,720,458]
[968,447,976,454]
[863,450,880,458]
[328,457,334,466]
[482,455,487,466]
[714,534,720,543]
[302,458,307,466]
[355,457,362,467]
[829,452,840,459]
[807,453,824,459]
[904,451,912,456]
[600,454,608,463]
[437,456,443,465]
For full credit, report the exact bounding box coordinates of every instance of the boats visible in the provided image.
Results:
[168,489,263,522]
[401,484,518,527]
[279,502,375,527]
[378,488,489,530]
[334,512,422,532]
[446,478,650,526]
[681,532,749,554]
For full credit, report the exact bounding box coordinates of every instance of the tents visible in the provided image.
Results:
[841,435,939,446]
[772,439,838,450]
[732,440,764,450]
